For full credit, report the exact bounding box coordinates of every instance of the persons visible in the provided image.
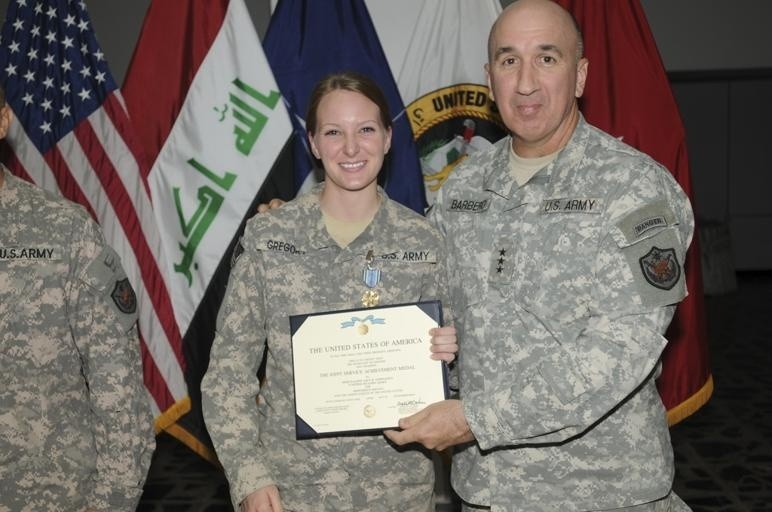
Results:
[256,1,696,511]
[199,69,460,510]
[0,80,158,511]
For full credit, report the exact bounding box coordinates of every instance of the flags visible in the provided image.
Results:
[118,0,298,464]
[0,1,192,432]
[366,1,504,215]
[556,0,715,427]
[261,0,426,215]
